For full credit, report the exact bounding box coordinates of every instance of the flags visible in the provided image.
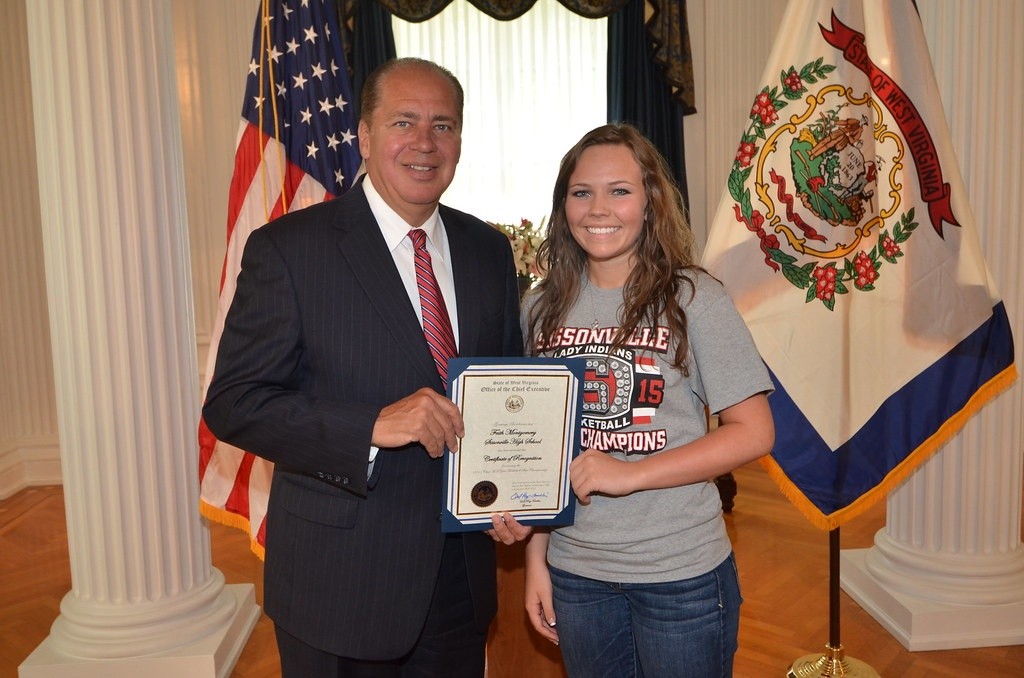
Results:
[199,0,397,562]
[700,0,1019,531]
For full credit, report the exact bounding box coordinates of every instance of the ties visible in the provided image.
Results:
[406,229,459,392]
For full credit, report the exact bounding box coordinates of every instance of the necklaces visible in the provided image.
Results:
[590,282,624,330]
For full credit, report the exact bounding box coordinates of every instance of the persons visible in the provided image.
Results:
[203,57,533,678]
[520,125,775,678]
